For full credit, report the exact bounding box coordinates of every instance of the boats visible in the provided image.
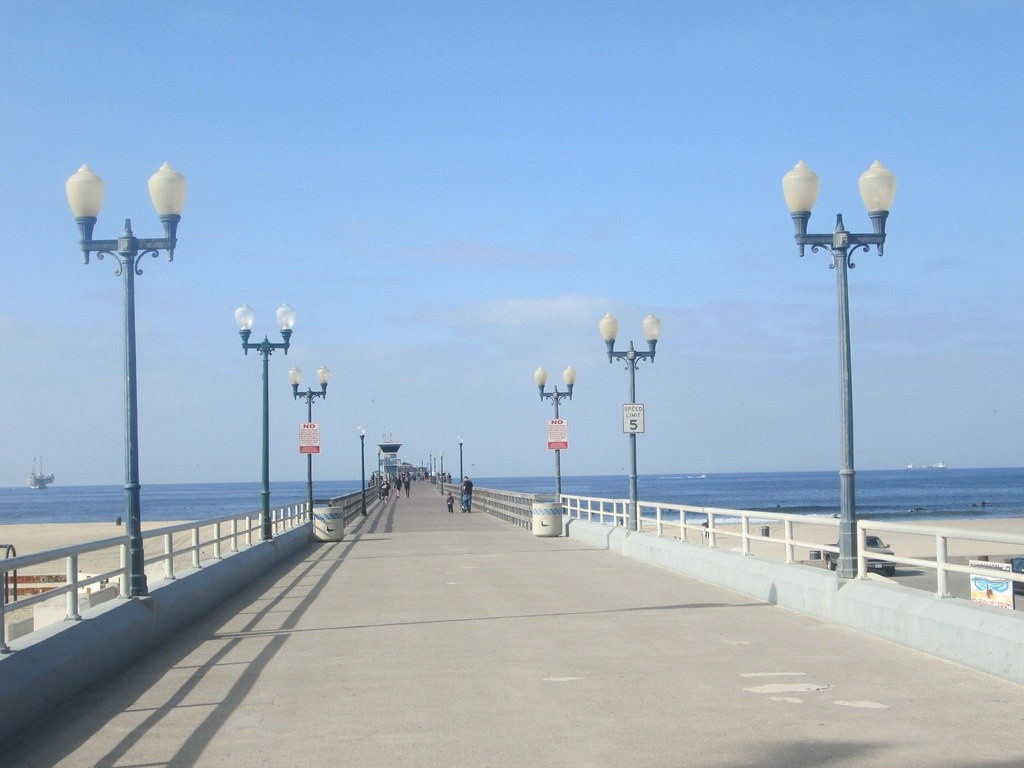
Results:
[931,461,947,469]
[908,464,913,470]
[25,454,55,490]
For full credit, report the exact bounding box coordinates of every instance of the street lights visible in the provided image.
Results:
[376,445,382,501]
[599,311,662,529]
[439,451,444,496]
[534,364,576,503]
[782,158,896,578]
[356,426,367,515]
[457,435,464,506]
[429,450,433,474]
[65,162,190,596]
[433,455,437,474]
[288,365,330,520]
[232,300,298,539]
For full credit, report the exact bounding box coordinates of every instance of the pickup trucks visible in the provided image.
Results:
[821,535,897,575]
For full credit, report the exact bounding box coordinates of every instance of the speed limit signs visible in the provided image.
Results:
[623,405,646,433]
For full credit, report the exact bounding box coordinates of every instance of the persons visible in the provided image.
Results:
[619,517,623,525]
[394,476,401,498]
[462,476,473,513]
[703,519,709,538]
[405,477,410,498]
[447,493,454,513]
[432,471,436,476]
[402,471,429,483]
[380,479,390,505]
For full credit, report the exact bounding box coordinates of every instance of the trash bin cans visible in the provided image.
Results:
[762,526,769,537]
[312,507,344,541]
[116,517,121,526]
[533,502,563,537]
[809,551,821,559]
